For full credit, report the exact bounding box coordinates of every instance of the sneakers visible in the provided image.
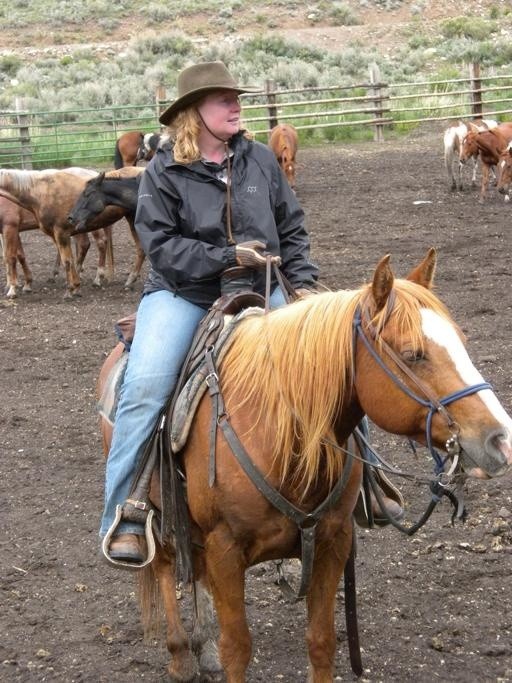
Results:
[109,534,148,562]
[369,492,404,523]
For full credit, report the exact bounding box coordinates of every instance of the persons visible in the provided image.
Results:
[98,58,404,566]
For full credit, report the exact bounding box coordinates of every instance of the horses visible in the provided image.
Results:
[444,119,512,207]
[267,124,299,189]
[114,132,172,170]
[0,164,146,303]
[95,246,511,683]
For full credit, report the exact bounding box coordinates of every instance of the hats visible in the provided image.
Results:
[159,60,264,126]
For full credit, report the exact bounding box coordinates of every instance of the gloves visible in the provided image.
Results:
[235,240,282,271]
